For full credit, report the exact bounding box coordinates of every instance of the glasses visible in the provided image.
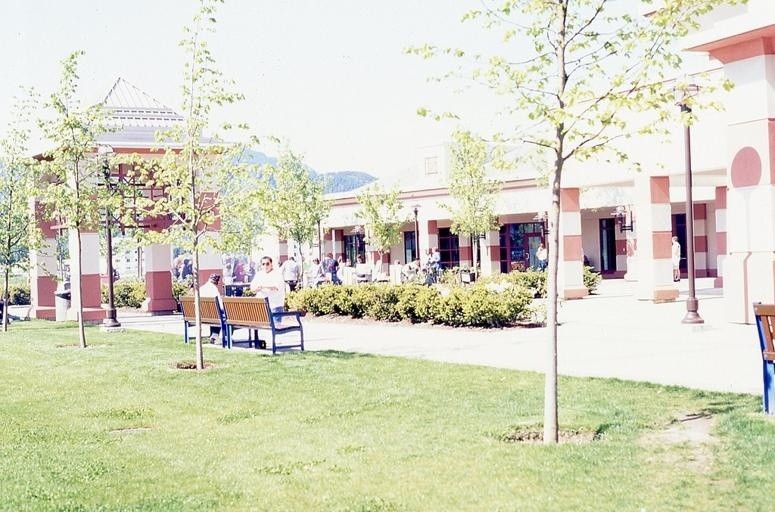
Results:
[262,262,269,265]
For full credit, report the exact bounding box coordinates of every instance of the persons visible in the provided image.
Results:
[176,259,193,281]
[535,243,548,272]
[0,293,31,325]
[199,275,224,344]
[672,237,681,282]
[222,248,442,323]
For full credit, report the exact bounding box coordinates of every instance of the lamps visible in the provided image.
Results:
[533,211,552,237]
[609,205,634,235]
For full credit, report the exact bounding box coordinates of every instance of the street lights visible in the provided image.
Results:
[676,95,705,324]
[413,208,421,262]
[97,145,124,328]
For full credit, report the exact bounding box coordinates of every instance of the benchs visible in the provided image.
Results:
[176,294,252,348]
[219,296,306,355]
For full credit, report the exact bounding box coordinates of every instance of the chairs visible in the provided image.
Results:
[753,300,775,413]
[222,268,437,297]
